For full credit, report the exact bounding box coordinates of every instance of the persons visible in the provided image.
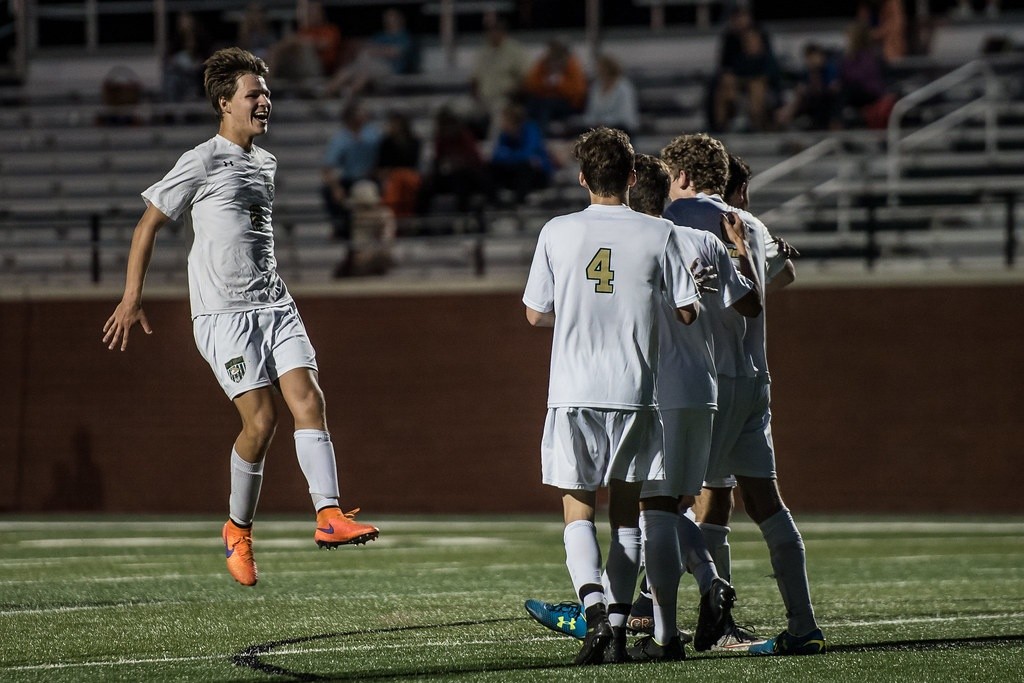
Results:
[101,46,378,587]
[520,125,826,662]
[165,0,1022,280]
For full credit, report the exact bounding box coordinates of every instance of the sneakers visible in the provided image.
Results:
[694,577,737,652]
[603,626,631,664]
[574,602,613,667]
[525,599,587,642]
[222,518,257,586]
[748,628,827,656]
[315,508,379,551]
[626,636,686,662]
[708,624,767,652]
[626,613,692,643]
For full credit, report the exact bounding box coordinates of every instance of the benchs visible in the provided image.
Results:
[0,53,1024,297]
